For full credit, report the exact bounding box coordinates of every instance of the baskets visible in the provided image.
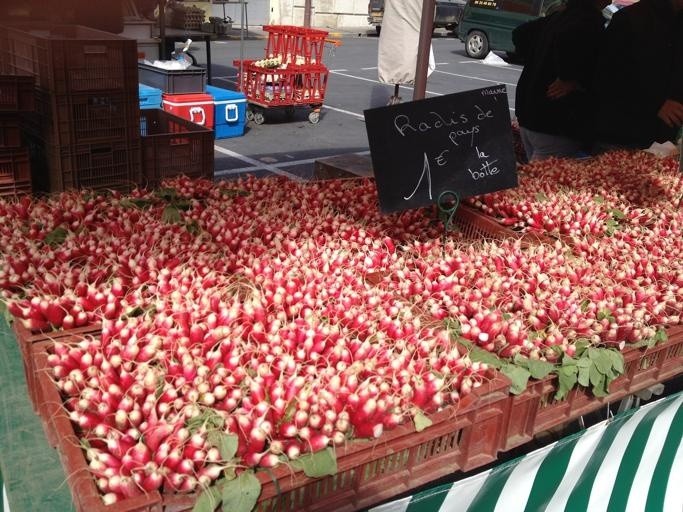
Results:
[10,170,682,511]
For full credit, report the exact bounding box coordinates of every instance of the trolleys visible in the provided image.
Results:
[233,25,328,124]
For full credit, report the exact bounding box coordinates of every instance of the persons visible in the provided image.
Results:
[601,0,682,148]
[510,0,612,161]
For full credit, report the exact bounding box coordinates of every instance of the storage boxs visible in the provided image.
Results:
[137,56,248,141]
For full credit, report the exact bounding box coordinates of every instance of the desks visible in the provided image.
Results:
[151,26,265,85]
[0,142,683,511]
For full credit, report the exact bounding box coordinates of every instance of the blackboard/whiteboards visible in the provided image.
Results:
[363,84,518,214]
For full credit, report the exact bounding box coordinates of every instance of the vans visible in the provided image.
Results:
[367,0,466,39]
[456,0,562,59]
